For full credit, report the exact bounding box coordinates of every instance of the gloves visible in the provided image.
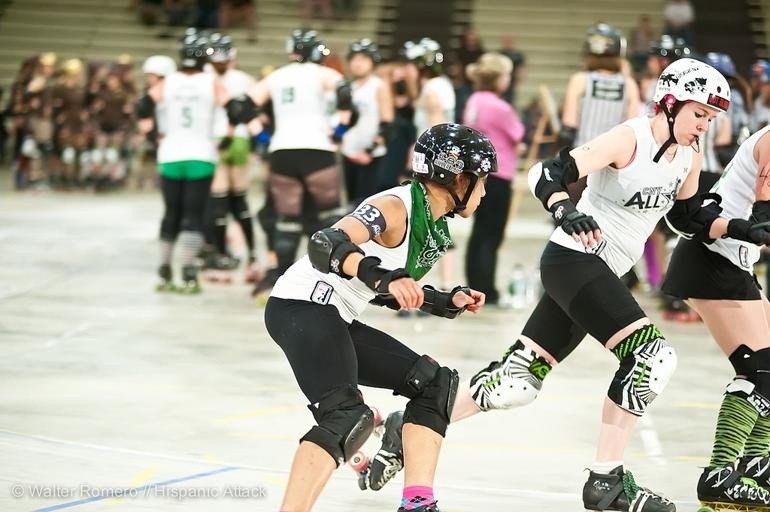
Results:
[745,220,770,247]
[550,199,602,236]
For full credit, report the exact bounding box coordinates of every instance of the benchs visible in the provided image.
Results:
[448,1,675,116]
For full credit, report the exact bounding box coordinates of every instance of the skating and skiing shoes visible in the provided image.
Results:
[736,455,770,486]
[697,461,770,505]
[582,465,676,512]
[156,264,175,292]
[251,266,289,304]
[246,258,261,282]
[349,406,404,490]
[204,252,238,283]
[179,263,200,294]
[663,295,699,323]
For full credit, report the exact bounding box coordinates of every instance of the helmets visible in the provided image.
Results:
[287,28,329,64]
[705,51,736,77]
[412,123,498,185]
[399,37,444,77]
[177,27,214,70]
[143,56,176,80]
[654,58,732,114]
[649,34,691,58]
[586,21,627,58]
[207,32,235,63]
[347,38,381,64]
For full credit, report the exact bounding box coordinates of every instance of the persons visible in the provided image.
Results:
[356,55,770,512]
[556,21,770,325]
[658,119,770,512]
[261,122,499,512]
[9,51,158,193]
[134,26,528,308]
